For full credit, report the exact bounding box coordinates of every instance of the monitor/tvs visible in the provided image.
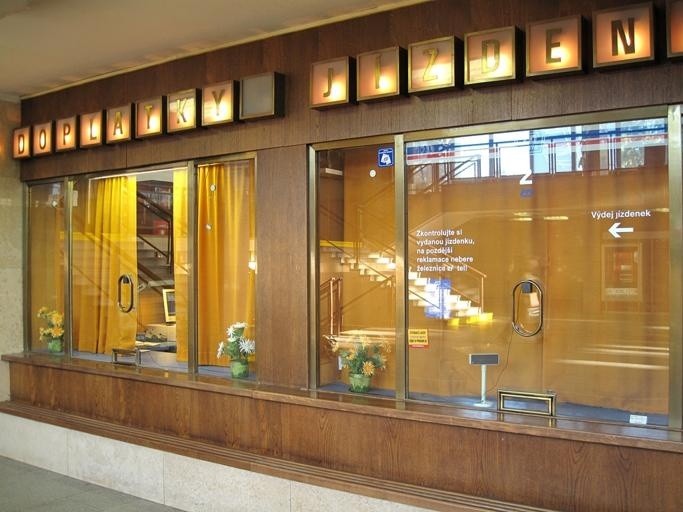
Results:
[162,288,176,326]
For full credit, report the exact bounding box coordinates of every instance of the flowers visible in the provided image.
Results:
[34,305,67,343]
[213,323,258,360]
[323,333,390,374]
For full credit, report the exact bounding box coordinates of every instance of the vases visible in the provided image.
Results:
[46,335,66,354]
[227,356,252,378]
[346,369,374,395]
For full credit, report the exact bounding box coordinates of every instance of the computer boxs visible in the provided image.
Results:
[146,324,177,342]
